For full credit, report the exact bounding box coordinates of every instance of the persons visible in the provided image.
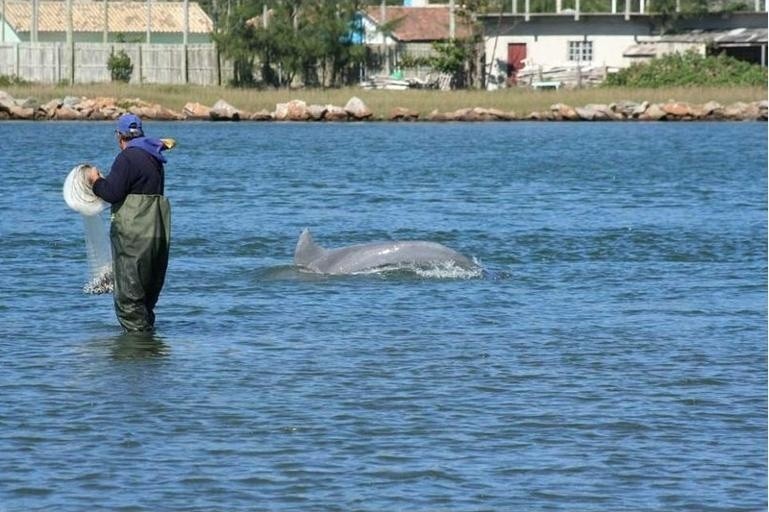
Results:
[85,114,172,341]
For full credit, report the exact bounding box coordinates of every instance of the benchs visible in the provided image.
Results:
[531,81,562,90]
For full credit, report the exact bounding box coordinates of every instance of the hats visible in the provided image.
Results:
[114,110,144,134]
